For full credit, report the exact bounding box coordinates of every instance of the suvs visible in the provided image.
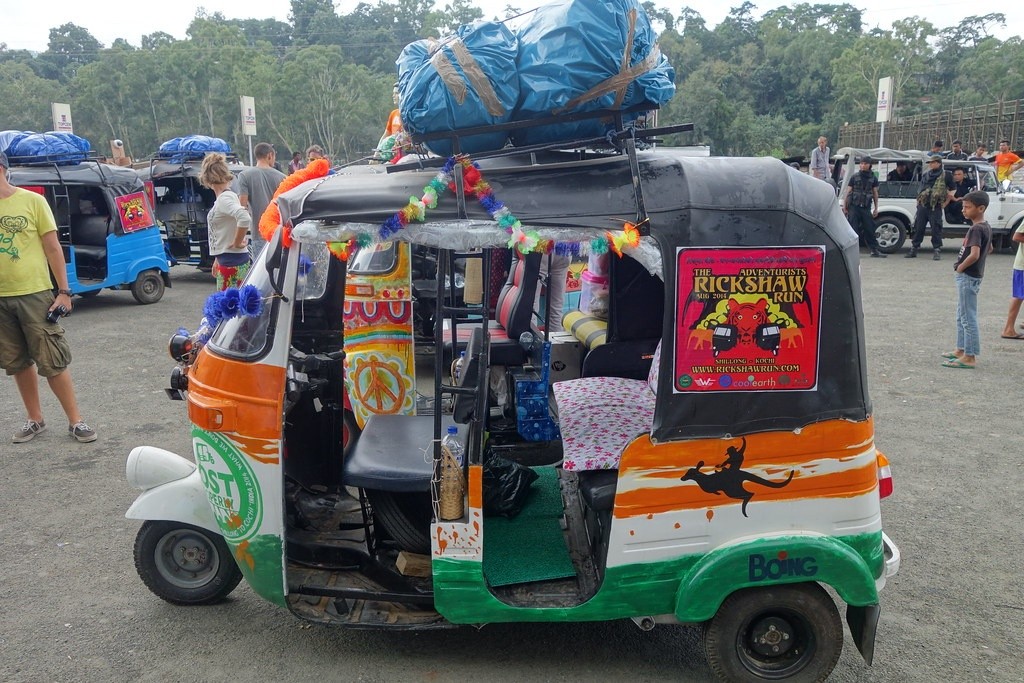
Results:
[830,146,1023,254]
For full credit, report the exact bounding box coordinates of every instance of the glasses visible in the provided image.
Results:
[306,157,322,162]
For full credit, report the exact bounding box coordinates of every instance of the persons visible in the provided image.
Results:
[811,136,831,179]
[307,145,337,175]
[967,142,987,161]
[531,241,580,333]
[927,141,945,156]
[199,154,250,293]
[942,191,992,369]
[993,141,1024,183]
[905,156,957,260]
[0,152,97,442]
[948,140,968,159]
[945,168,972,224]
[289,151,305,175]
[1001,220,1024,340]
[238,142,287,263]
[380,82,402,148]
[887,162,913,181]
[843,156,886,257]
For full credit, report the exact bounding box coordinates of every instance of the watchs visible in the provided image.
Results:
[59,289,72,296]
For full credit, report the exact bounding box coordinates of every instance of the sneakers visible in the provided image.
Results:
[10,419,48,443]
[68,421,97,442]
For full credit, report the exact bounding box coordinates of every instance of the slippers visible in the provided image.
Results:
[942,351,959,359]
[1001,333,1024,339]
[942,358,976,369]
[1019,322,1024,329]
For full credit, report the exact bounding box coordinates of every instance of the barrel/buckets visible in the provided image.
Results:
[189,192,202,202]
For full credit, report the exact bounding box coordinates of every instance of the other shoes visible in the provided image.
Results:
[932,248,942,260]
[905,247,922,258]
[868,249,890,257]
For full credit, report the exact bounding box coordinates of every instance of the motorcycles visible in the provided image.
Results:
[124,104,900,683]
[2,152,252,306]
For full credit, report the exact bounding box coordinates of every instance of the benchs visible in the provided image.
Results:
[344,326,495,492]
[59,215,116,260]
[441,243,542,366]
[553,338,662,505]
[156,201,209,237]
[561,308,608,349]
[877,180,922,199]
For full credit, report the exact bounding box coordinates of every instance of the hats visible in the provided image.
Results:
[859,155,874,166]
[0,151,9,169]
[924,155,948,165]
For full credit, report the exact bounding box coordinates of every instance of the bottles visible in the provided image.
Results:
[453,351,466,418]
[435,271,464,288]
[519,331,533,351]
[175,195,184,203]
[439,426,465,498]
